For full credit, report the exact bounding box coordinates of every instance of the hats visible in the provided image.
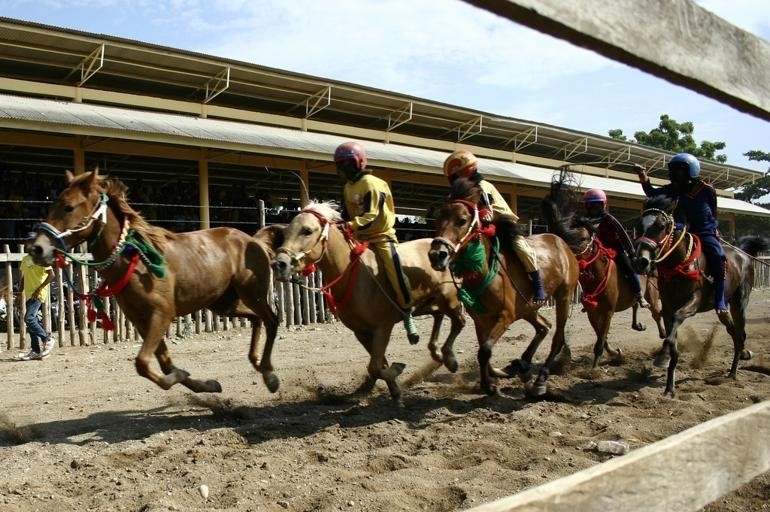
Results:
[22,231,40,241]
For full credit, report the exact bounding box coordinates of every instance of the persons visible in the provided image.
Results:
[334,143,420,345]
[125,176,301,231]
[0,167,67,246]
[443,151,545,302]
[527,216,545,234]
[15,231,57,362]
[633,151,729,315]
[394,217,437,241]
[584,190,652,308]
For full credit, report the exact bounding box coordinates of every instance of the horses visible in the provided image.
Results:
[631,193,769,400]
[270,200,466,410]
[27,166,286,394]
[425,164,582,400]
[566,215,667,378]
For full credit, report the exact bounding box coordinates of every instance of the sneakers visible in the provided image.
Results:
[21,349,43,361]
[42,336,55,356]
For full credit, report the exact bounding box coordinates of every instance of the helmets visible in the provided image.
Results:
[580,188,607,211]
[668,153,700,179]
[334,142,367,178]
[443,150,479,178]
